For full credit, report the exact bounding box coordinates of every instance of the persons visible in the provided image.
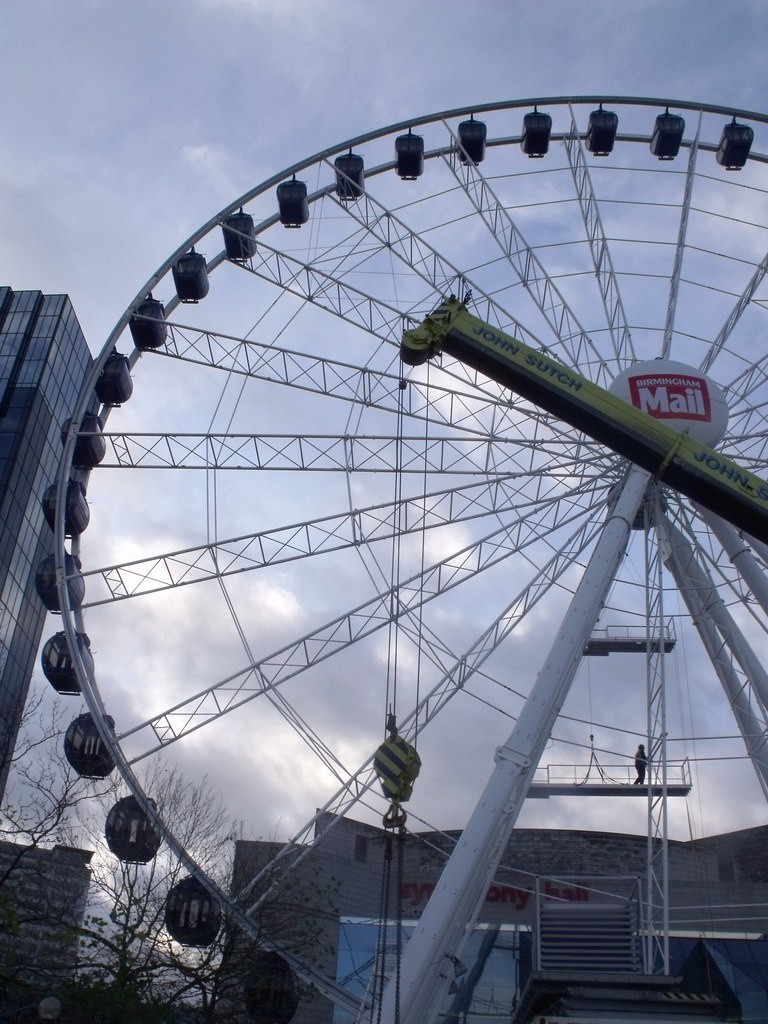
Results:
[634,744,646,785]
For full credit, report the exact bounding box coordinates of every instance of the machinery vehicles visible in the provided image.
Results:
[400,292,768,542]
